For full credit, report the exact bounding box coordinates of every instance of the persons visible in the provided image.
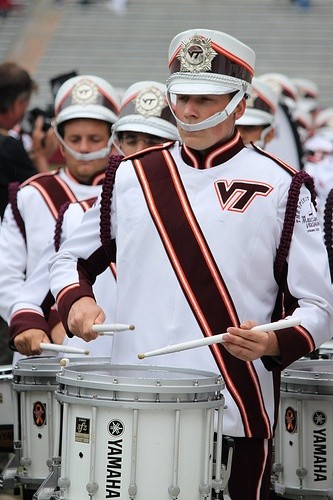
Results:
[0,74,121,367]
[0,60,128,221]
[232,66,333,235]
[5,82,181,356]
[48,28,333,500]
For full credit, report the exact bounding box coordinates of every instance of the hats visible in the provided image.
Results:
[109,81,182,158]
[165,28,256,131]
[51,75,120,161]
[234,72,333,148]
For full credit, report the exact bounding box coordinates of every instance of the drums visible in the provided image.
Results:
[9,356,114,490]
[0,363,15,426]
[269,356,333,500]
[50,362,227,500]
